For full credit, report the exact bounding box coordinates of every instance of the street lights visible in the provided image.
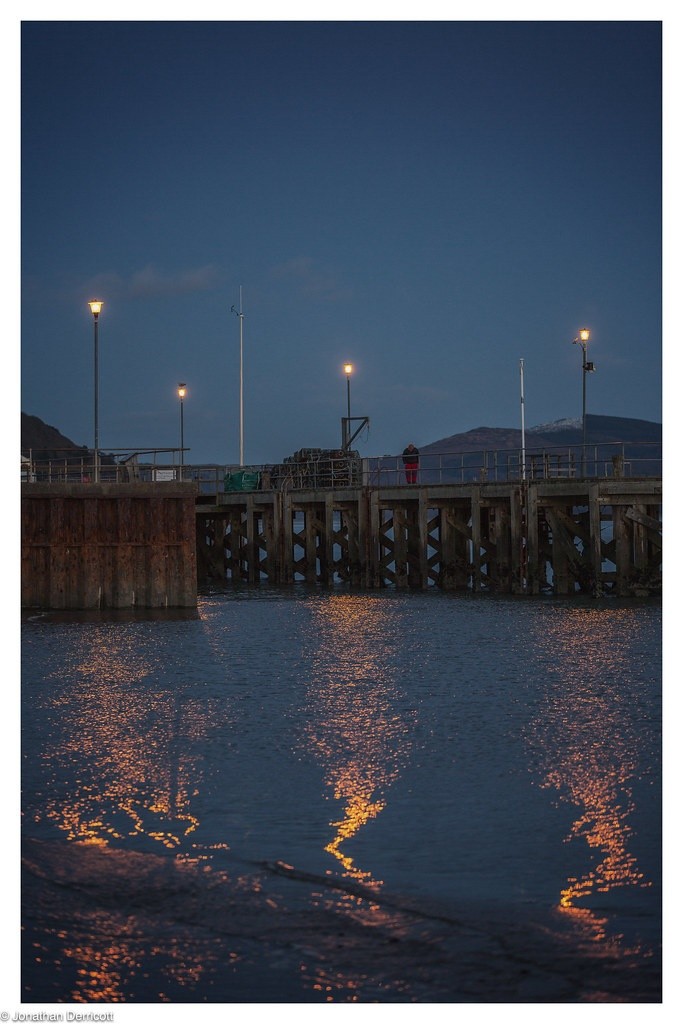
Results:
[580,328,590,476]
[178,383,186,465]
[345,364,352,451]
[89,299,103,481]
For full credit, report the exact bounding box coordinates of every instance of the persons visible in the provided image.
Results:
[402,445,420,484]
